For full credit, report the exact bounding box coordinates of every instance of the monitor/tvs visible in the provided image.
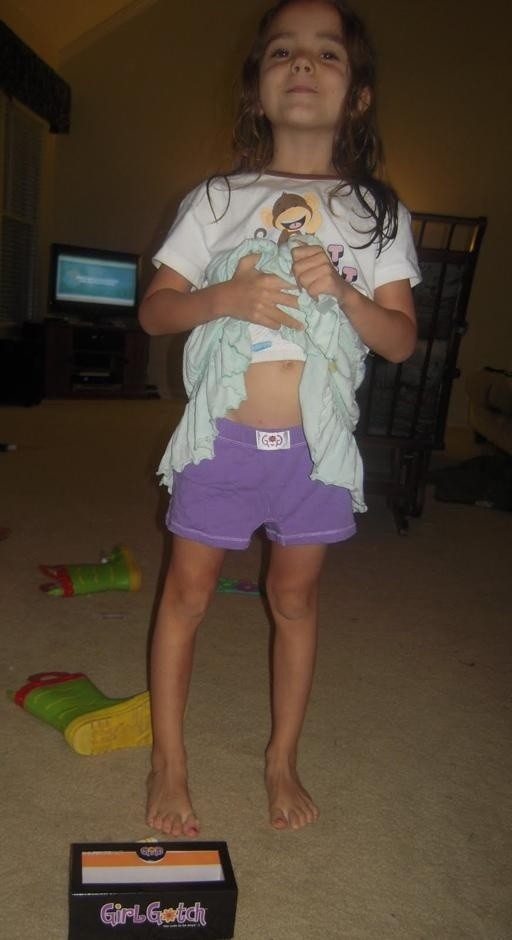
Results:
[48,243,139,326]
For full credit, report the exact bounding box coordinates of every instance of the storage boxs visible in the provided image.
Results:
[66,842,238,938]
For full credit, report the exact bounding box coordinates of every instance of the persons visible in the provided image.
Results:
[137,1,424,838]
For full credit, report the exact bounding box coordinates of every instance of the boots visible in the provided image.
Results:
[12,672,154,757]
[38,544,141,597]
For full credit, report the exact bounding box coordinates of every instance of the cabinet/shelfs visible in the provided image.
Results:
[43,318,148,400]
